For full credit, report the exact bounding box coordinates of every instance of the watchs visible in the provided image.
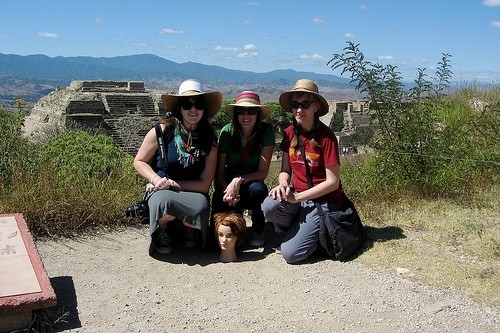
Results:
[237,175,245,185]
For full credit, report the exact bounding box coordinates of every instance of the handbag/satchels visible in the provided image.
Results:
[319,189,369,262]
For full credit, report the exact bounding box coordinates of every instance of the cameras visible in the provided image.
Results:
[125,200,150,224]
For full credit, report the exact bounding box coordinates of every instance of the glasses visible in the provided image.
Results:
[236,107,259,115]
[288,97,315,109]
[180,97,206,110]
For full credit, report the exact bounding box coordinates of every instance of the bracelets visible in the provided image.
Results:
[151,174,158,184]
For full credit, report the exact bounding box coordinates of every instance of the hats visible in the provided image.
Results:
[161,79,224,120]
[226,90,271,122]
[279,78,329,117]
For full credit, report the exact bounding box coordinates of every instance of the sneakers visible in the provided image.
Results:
[249,224,267,246]
[154,227,174,255]
[183,227,198,247]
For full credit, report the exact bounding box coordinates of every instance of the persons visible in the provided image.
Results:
[210,91,276,246]
[260,78,340,264]
[132,77,223,255]
[214,213,244,262]
